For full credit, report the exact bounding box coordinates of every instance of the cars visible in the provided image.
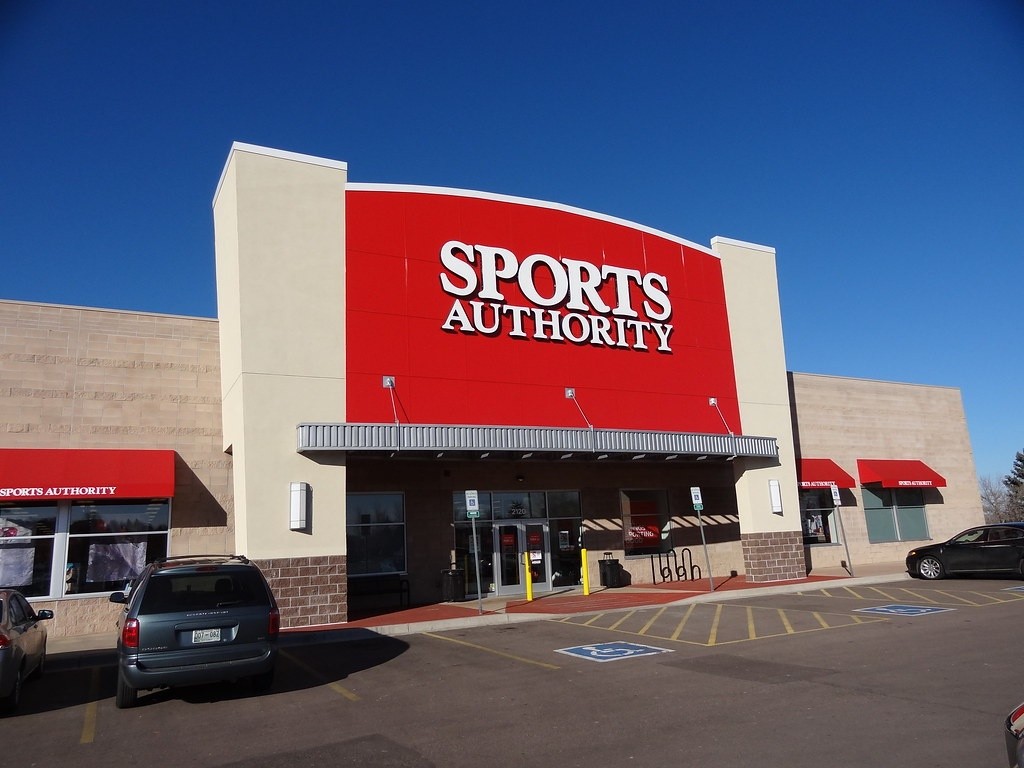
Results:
[904,522,1024,580]
[0,588,54,714]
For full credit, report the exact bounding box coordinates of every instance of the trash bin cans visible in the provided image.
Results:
[441,568,466,602]
[597,559,619,588]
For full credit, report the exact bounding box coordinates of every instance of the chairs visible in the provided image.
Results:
[214,578,233,593]
[1004,530,1012,536]
[993,532,1000,540]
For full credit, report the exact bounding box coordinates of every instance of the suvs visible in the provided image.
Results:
[109,554,280,708]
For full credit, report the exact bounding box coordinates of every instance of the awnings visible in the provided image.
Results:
[857,460,946,488]
[0,449,176,504]
[795,459,855,488]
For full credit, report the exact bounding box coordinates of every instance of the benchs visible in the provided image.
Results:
[348,579,411,609]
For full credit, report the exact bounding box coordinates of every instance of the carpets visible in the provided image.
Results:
[506,592,714,615]
[279,604,501,632]
[621,574,859,592]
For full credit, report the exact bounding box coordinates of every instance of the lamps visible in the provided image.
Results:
[143,577,173,605]
[770,480,783,513]
[479,451,490,459]
[696,454,707,461]
[631,452,646,460]
[435,450,444,458]
[388,450,395,458]
[520,451,533,459]
[290,481,307,531]
[664,453,679,461]
[515,474,526,483]
[723,455,734,462]
[595,453,608,460]
